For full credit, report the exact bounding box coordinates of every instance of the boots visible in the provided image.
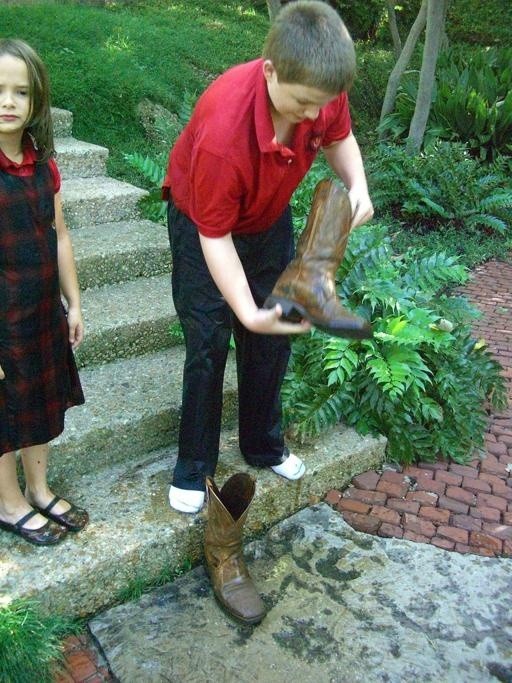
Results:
[262,176,375,338]
[201,471,266,622]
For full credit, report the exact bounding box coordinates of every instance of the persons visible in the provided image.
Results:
[1,37,88,545]
[159,0,375,512]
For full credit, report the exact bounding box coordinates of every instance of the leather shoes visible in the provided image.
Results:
[0,508,67,544]
[39,494,88,531]
